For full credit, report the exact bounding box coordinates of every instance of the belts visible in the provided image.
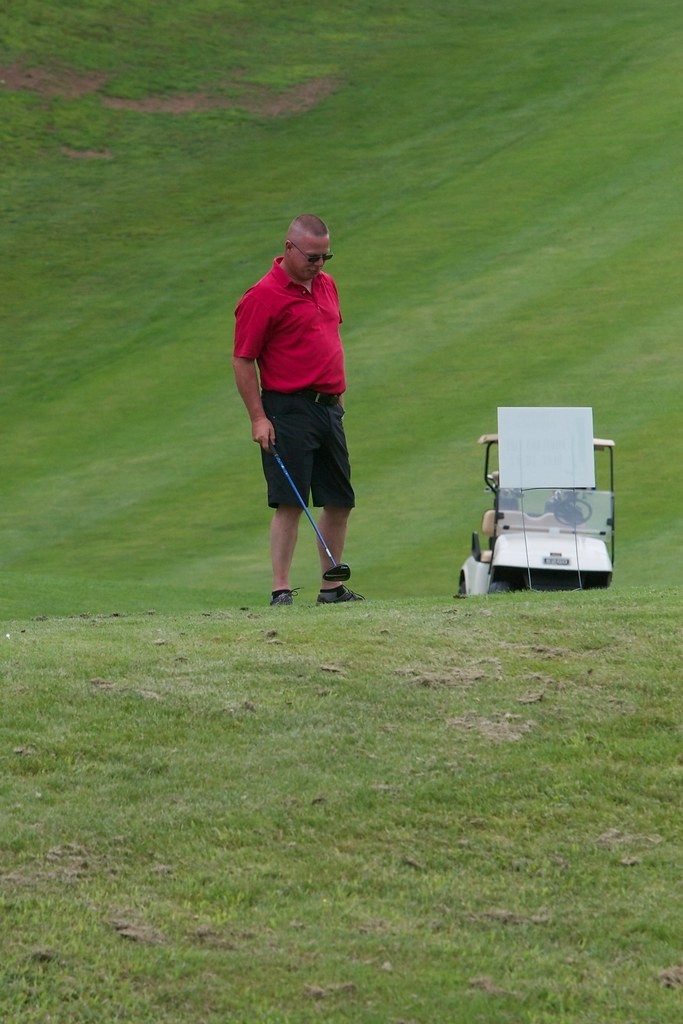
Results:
[299,388,341,409]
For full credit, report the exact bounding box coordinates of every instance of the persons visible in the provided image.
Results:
[231,214,366,605]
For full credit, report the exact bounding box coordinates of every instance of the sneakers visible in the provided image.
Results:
[269,586,301,605]
[316,583,366,603]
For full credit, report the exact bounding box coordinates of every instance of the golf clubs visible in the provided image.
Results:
[266,438,352,581]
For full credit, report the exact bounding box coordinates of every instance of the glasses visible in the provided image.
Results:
[290,240,333,263]
